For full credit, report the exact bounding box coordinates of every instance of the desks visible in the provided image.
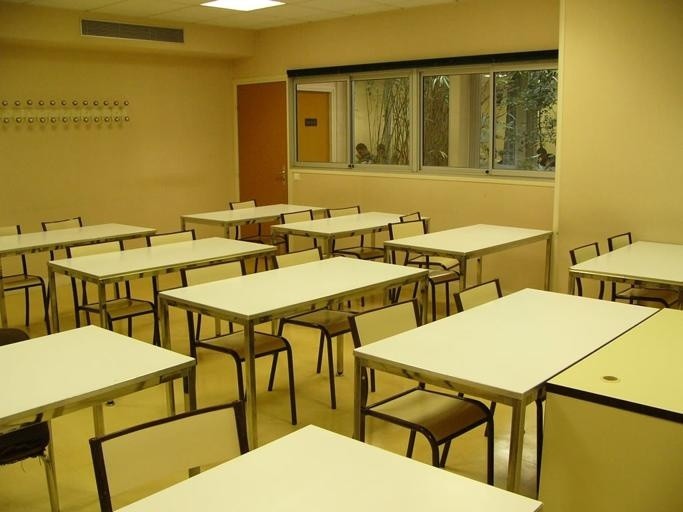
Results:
[568,240,683,295]
[384,223,553,325]
[112,424,545,512]
[0,222,158,328]
[46,236,278,329]
[1,324,197,436]
[180,204,329,239]
[270,212,431,260]
[159,256,430,450]
[352,287,660,494]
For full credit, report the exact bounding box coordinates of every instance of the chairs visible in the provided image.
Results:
[0,224,51,335]
[569,242,605,300]
[326,206,384,260]
[395,212,459,303]
[347,298,494,487]
[89,399,249,512]
[146,228,234,346]
[268,245,376,409]
[453,279,543,436]
[180,259,297,426]
[41,216,120,322]
[229,198,261,240]
[608,231,679,309]
[280,209,317,254]
[66,238,161,347]
[0,328,61,511]
[388,219,460,322]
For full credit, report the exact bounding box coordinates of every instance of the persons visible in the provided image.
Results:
[355,143,373,163]
[536,146,555,167]
